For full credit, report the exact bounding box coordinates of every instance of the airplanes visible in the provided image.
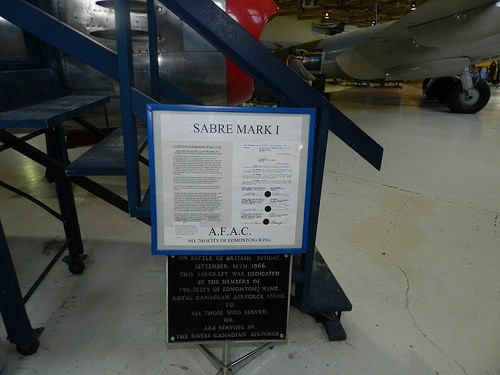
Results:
[280,1,500,114]
